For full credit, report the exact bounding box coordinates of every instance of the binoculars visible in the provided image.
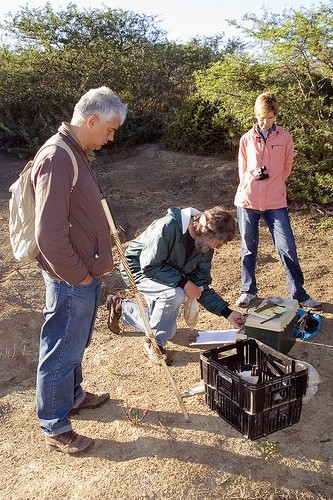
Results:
[253,165,269,180]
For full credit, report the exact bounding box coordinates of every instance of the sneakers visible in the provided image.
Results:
[105,294,123,335]
[72,391,110,411]
[45,430,95,456]
[235,292,259,308]
[299,296,323,310]
[143,338,173,366]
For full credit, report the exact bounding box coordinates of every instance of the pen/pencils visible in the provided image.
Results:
[260,314,279,324]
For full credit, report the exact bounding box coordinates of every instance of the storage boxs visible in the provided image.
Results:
[245,295,300,354]
[199,337,309,441]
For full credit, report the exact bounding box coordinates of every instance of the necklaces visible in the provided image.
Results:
[59,131,106,198]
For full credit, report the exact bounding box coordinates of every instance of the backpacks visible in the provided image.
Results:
[8,139,79,263]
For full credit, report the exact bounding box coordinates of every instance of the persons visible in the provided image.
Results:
[29,88,126,456]
[234,91,322,310]
[106,206,246,366]
